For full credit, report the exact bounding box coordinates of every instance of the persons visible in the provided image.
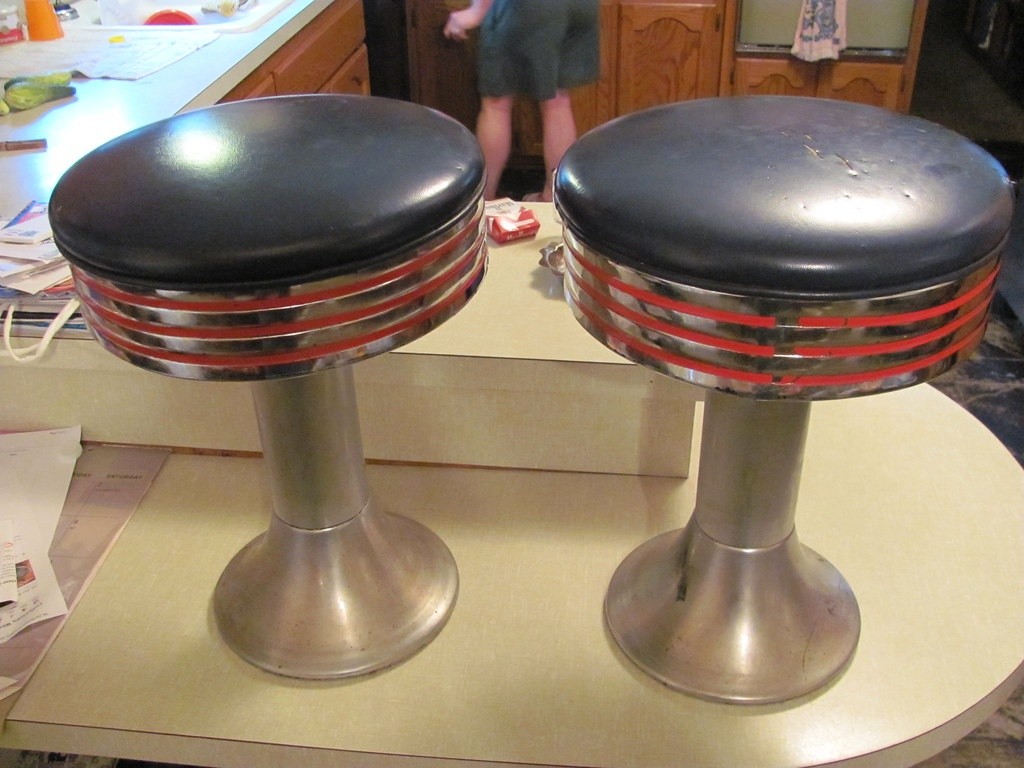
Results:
[443,0,601,203]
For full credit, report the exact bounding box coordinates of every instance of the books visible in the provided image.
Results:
[0,199,95,340]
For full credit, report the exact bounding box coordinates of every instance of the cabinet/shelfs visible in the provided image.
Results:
[407,0,929,169]
[217,0,371,104]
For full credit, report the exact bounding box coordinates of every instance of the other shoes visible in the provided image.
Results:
[523,193,541,201]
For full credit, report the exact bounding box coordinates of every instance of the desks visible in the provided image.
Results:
[0,200,1024,768]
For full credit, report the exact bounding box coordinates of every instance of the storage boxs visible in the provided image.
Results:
[484,198,541,243]
